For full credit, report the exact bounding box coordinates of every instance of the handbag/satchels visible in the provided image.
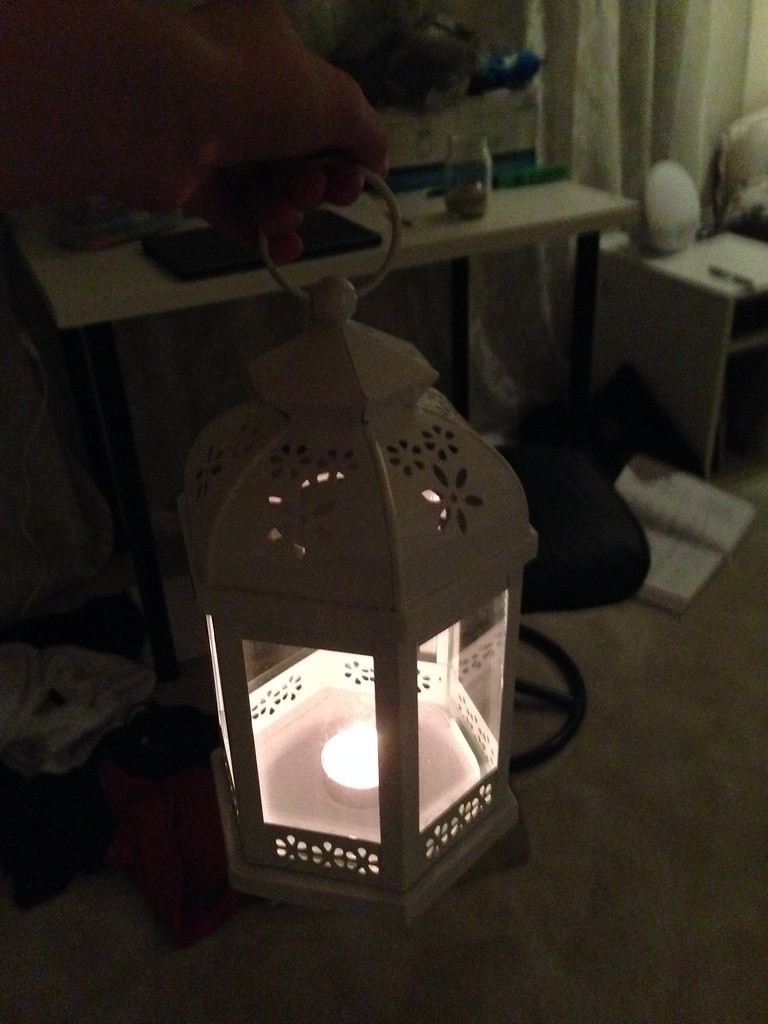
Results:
[105,702,246,951]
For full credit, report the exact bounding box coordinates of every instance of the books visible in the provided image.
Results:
[609,450,758,612]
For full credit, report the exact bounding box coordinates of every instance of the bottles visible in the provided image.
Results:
[441,132,492,218]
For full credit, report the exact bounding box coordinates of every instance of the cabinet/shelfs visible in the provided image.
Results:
[576,233,768,479]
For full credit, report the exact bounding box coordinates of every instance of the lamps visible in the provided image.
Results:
[184,180,534,926]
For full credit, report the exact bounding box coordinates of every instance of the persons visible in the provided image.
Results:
[0,0,393,263]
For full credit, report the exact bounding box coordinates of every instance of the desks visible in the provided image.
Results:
[8,177,639,700]
